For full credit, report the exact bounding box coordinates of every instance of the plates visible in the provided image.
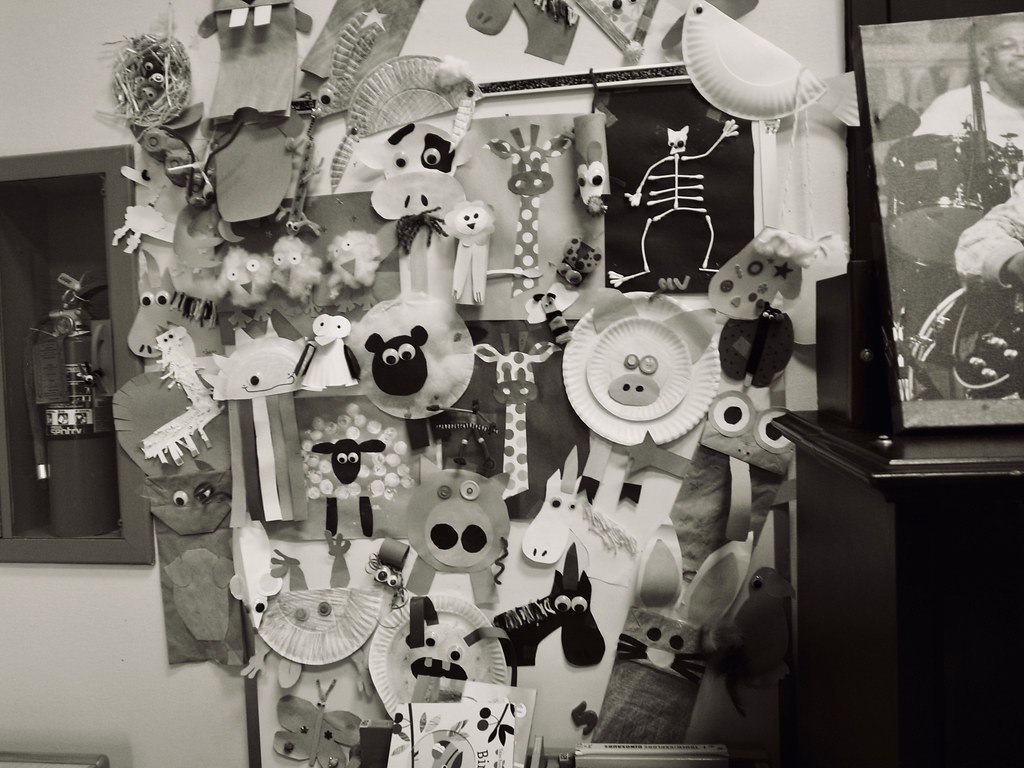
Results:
[346,54,485,139]
[680,0,827,121]
[256,589,382,666]
[587,316,692,422]
[368,594,507,720]
[358,294,474,420]
[561,292,721,448]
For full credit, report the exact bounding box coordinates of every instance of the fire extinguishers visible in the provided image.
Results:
[30,269,120,538]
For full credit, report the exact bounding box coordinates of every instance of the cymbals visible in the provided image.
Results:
[864,92,922,144]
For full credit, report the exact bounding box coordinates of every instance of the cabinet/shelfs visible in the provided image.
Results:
[768,414,1023,767]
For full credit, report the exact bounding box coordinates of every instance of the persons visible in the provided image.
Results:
[911,23,1024,292]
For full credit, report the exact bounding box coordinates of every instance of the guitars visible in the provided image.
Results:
[953,283,1024,402]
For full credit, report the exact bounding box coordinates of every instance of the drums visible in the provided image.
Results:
[880,134,1012,401]
